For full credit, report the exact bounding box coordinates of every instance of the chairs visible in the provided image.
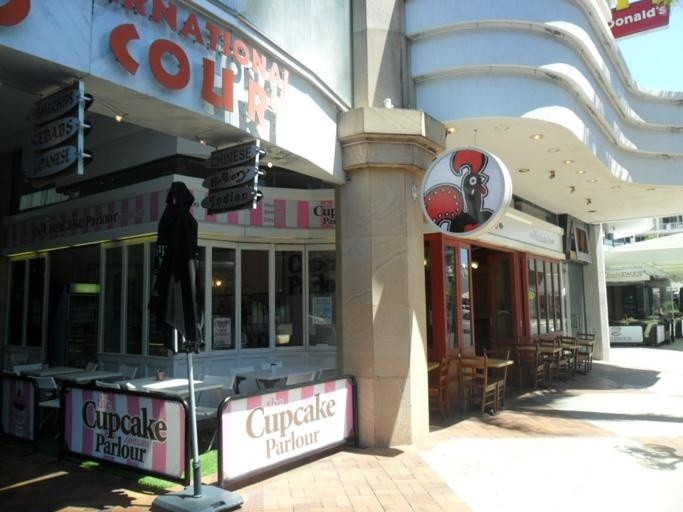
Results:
[427,332,596,421]
[0,350,336,454]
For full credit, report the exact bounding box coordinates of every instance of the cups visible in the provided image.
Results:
[157,371,163,379]
